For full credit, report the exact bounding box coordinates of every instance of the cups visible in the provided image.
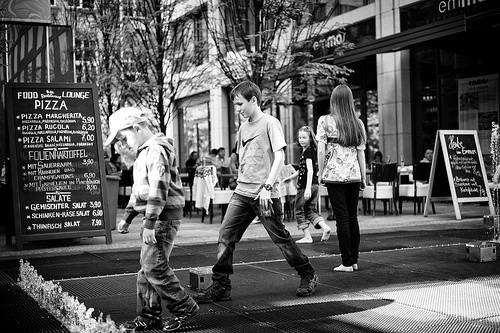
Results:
[385,154,392,165]
[400,155,405,171]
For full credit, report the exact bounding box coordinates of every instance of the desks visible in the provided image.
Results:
[366,168,411,173]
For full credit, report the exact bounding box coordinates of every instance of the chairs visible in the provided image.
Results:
[118,162,436,225]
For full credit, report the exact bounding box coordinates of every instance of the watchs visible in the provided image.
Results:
[262,183,273,191]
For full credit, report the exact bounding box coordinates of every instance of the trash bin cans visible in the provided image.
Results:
[106,175,122,230]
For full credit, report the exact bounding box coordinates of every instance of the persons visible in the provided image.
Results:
[194,81,321,303]
[281,125,332,243]
[186,145,242,191]
[105,105,201,332]
[110,153,129,171]
[315,86,368,272]
[419,148,434,163]
[369,141,383,162]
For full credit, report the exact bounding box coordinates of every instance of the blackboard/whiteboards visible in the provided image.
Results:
[4,81,112,243]
[425,129,494,203]
